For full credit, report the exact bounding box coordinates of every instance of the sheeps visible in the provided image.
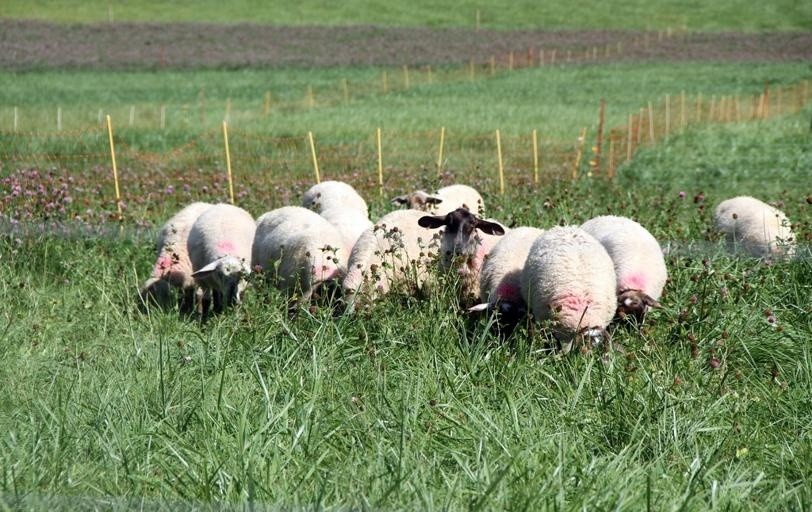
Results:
[144,179,546,337]
[578,213,668,334]
[519,224,618,355]
[715,195,798,267]
